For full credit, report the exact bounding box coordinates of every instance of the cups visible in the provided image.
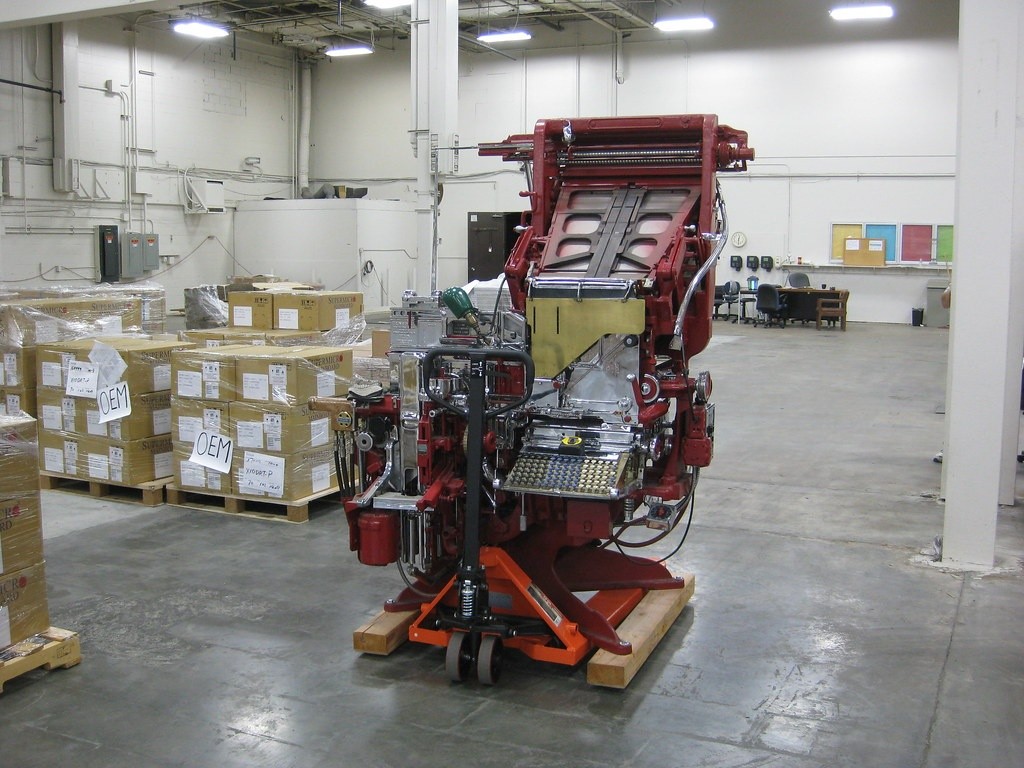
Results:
[797,256,802,264]
[822,284,826,289]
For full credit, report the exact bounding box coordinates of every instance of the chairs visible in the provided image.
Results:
[754,283,787,328]
[788,273,813,323]
[816,290,850,332]
[712,281,740,323]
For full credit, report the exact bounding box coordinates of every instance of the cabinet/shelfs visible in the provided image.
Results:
[926,279,950,327]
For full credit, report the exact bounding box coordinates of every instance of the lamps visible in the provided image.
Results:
[476,0,531,42]
[653,0,714,32]
[325,24,376,56]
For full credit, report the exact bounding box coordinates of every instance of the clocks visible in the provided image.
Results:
[731,232,747,247]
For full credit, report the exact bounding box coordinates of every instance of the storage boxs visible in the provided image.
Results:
[1,276,421,695]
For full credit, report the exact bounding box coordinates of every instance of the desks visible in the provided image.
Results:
[774,287,840,328]
[723,287,757,316]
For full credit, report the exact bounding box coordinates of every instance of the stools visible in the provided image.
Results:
[741,298,756,323]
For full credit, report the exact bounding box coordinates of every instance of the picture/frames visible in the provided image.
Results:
[830,223,953,265]
